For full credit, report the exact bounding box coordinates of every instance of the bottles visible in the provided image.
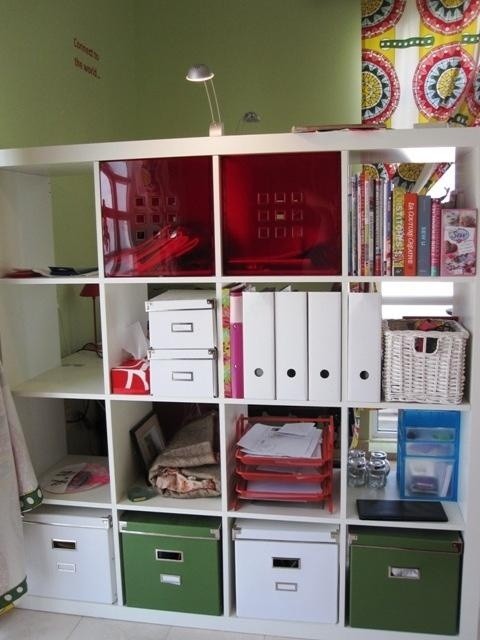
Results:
[347,449,391,489]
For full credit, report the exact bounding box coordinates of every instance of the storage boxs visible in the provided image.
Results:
[144,289,218,349]
[346,526,462,635]
[228,516,338,620]
[149,348,218,399]
[111,355,150,394]
[23,505,118,605]
[114,511,222,616]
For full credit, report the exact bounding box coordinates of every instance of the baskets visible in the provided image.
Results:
[382,318,470,405]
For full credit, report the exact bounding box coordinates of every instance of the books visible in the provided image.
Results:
[291,123,380,132]
[355,498,449,521]
[350,170,440,276]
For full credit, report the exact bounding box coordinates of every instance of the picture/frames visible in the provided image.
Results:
[130,410,168,485]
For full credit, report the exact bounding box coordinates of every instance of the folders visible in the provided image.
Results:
[229,285,382,403]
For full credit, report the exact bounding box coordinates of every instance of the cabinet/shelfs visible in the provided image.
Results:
[1,126,480,621]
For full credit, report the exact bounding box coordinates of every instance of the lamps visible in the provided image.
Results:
[187,64,224,136]
